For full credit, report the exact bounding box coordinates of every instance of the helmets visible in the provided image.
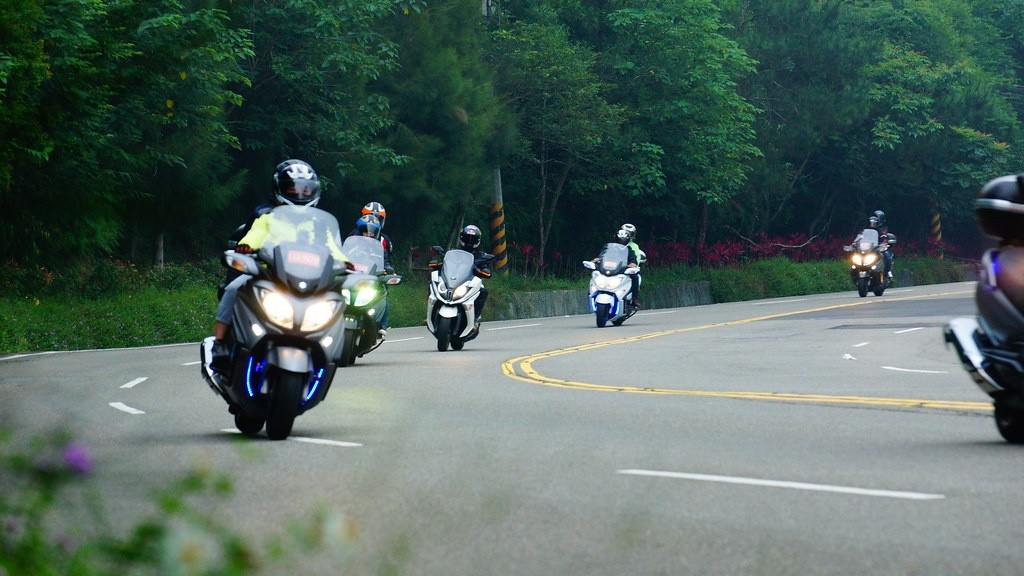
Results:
[874,210,884,222]
[615,229,630,245]
[866,216,879,229]
[459,225,481,248]
[362,203,387,228]
[357,214,381,238]
[274,158,321,207]
[621,223,637,238]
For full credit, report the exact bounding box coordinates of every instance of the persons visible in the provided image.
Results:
[348,202,397,336]
[851,211,894,279]
[588,223,642,307]
[211,160,356,361]
[424,225,491,332]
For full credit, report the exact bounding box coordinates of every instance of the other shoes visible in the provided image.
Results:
[630,301,641,308]
[378,328,386,336]
[212,341,228,361]
[888,271,893,278]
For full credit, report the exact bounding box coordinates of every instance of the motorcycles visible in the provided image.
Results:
[199,204,356,443]
[942,172,1024,447]
[845,229,898,299]
[340,237,401,367]
[583,245,643,328]
[427,244,495,353]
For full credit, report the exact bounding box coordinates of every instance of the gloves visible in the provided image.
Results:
[482,268,491,275]
[429,259,438,265]
[386,266,395,275]
